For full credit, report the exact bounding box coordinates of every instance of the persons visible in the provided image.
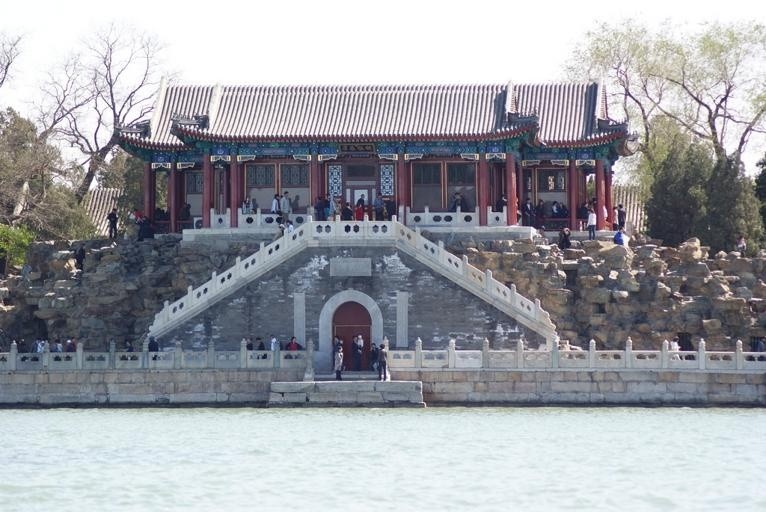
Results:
[148,336,159,360]
[134,208,143,223]
[19,262,33,284]
[241,196,256,223]
[267,334,283,360]
[757,335,766,361]
[107,208,118,239]
[329,334,388,382]
[75,241,86,271]
[15,333,78,362]
[271,192,291,224]
[246,336,254,359]
[253,337,267,359]
[178,202,192,222]
[121,339,137,360]
[450,192,468,212]
[284,336,306,359]
[516,197,627,250]
[670,334,682,361]
[316,192,397,222]
[496,195,508,211]
[737,235,748,258]
